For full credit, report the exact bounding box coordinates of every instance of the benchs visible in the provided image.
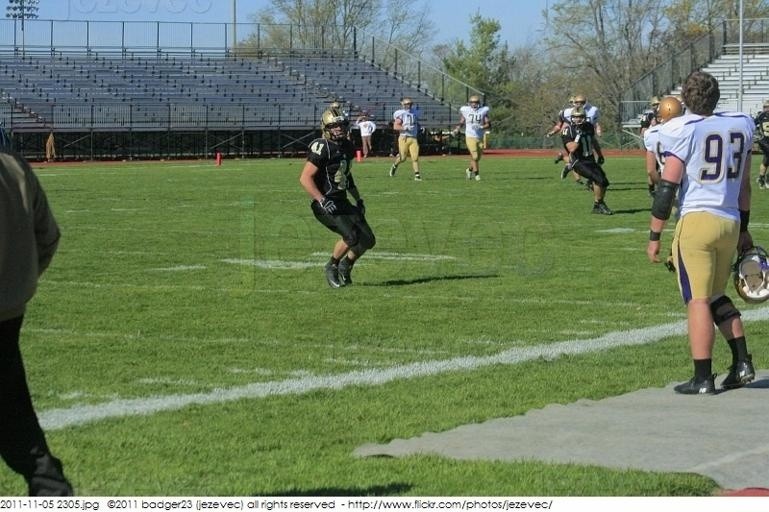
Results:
[620,41,769,131]
[0,44,472,131]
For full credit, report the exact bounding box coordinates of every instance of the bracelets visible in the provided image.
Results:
[738,209,752,233]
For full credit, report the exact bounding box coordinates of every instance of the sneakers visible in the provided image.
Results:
[758,174,769,190]
[574,178,584,185]
[324,260,340,288]
[465,168,481,181]
[583,183,594,193]
[390,153,400,157]
[554,150,565,164]
[338,261,352,285]
[560,163,571,179]
[674,376,719,395]
[414,175,423,181]
[591,200,613,215]
[389,163,398,177]
[720,358,756,389]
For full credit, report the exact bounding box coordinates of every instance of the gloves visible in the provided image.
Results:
[546,130,556,137]
[472,125,483,131]
[575,128,584,143]
[403,126,415,131]
[319,197,338,215]
[450,127,462,138]
[597,154,605,164]
[421,128,426,134]
[356,199,366,215]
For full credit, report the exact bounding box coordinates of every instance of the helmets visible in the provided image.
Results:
[320,109,349,141]
[568,94,586,104]
[401,98,413,105]
[330,101,340,108]
[571,107,587,125]
[469,95,481,110]
[650,93,683,120]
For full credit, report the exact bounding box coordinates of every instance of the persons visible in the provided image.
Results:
[450,94,492,182]
[1,148,74,496]
[754,99,769,191]
[644,70,755,398]
[388,97,427,183]
[546,94,683,274]
[298,102,377,292]
[354,111,379,160]
